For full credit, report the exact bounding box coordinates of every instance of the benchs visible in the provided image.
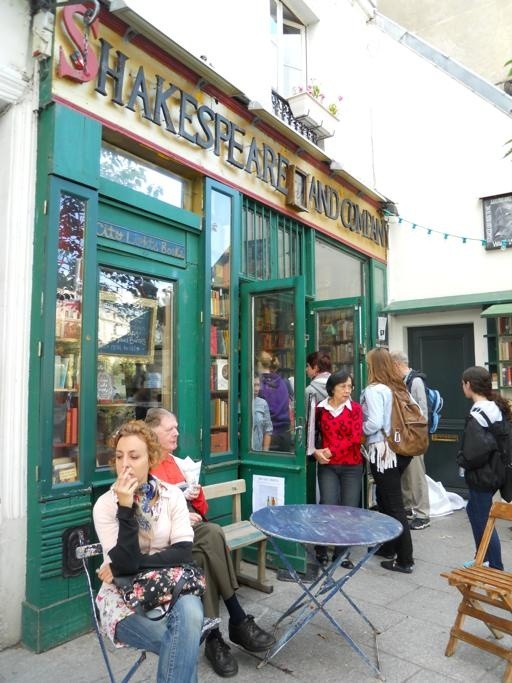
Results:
[201,479,274,604]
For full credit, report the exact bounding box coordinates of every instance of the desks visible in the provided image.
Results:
[250,504,404,683]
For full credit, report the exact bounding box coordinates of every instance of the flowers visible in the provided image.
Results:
[293,78,345,114]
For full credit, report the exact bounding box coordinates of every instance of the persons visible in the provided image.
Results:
[389,349,431,531]
[145,407,275,677]
[252,374,273,451]
[359,346,420,573]
[458,367,512,572]
[306,369,363,569]
[95,420,205,683]
[256,352,293,451]
[304,350,352,420]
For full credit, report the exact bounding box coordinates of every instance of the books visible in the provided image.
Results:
[53,299,80,339]
[65,411,72,443]
[54,353,80,390]
[499,338,512,387]
[317,308,355,369]
[50,455,78,484]
[256,298,296,377]
[210,263,229,454]
[71,407,77,445]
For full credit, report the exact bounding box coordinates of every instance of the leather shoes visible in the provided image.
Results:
[228,615,277,652]
[367,545,396,559]
[380,559,414,574]
[204,634,239,677]
[332,553,355,569]
[315,552,328,566]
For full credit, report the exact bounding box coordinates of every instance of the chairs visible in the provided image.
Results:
[75,541,220,682]
[440,500,511,683]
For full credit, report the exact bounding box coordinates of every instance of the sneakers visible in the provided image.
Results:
[463,559,478,568]
[404,509,413,518]
[410,518,432,530]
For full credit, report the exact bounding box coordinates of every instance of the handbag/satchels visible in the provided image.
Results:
[307,420,323,463]
[119,561,207,612]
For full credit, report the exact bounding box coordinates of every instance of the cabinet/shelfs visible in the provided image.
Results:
[53,288,82,466]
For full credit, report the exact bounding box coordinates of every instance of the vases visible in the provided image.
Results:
[287,91,340,138]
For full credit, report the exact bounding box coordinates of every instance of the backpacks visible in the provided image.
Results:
[387,386,429,457]
[425,388,444,434]
[497,462,512,504]
